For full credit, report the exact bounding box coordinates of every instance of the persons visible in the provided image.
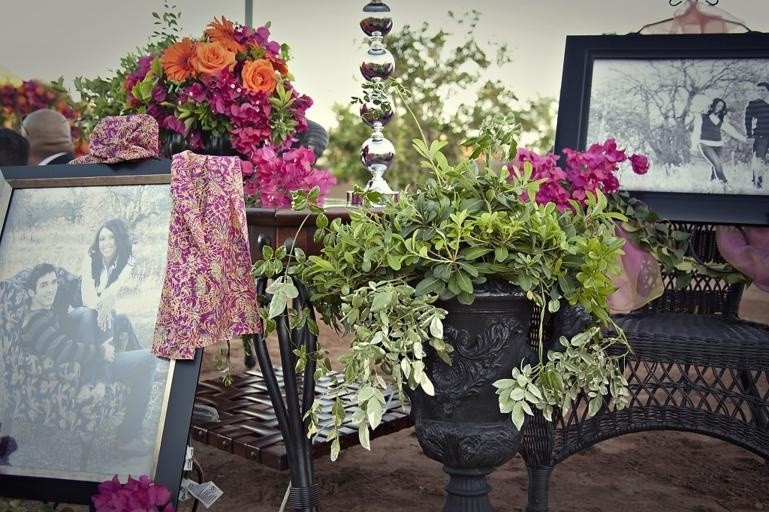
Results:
[687,96,749,183]
[75,217,142,332]
[19,109,77,165]
[1,130,33,168]
[14,262,167,454]
[744,82,769,189]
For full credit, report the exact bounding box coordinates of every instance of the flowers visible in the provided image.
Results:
[504,136,651,222]
[1,1,340,209]
[89,471,174,510]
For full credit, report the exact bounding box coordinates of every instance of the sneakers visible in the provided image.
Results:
[720,181,731,192]
[754,182,764,191]
[113,434,156,454]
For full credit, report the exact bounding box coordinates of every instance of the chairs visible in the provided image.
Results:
[514,226,769,512]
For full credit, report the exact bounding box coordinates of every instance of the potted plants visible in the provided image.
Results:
[248,74,629,512]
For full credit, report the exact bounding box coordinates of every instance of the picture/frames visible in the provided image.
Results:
[551,32,769,228]
[0,160,205,511]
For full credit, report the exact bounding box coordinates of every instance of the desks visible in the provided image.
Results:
[187,201,414,511]
